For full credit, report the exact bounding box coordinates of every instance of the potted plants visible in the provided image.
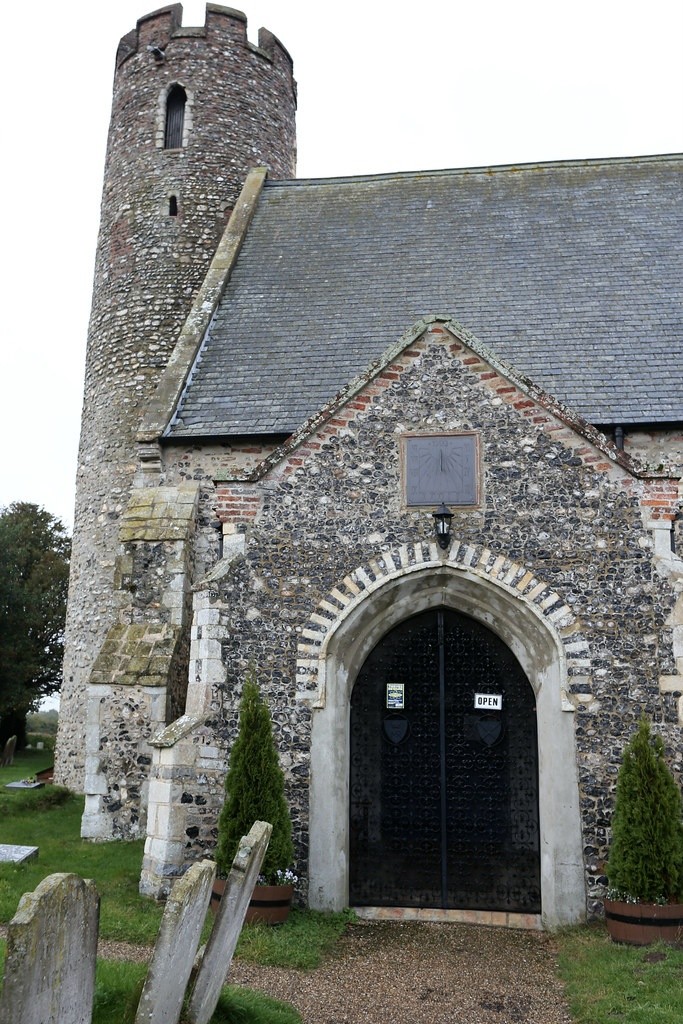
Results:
[602,712,683,945]
[212,681,293,925]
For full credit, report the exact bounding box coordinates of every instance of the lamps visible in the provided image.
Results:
[431,502,455,549]
